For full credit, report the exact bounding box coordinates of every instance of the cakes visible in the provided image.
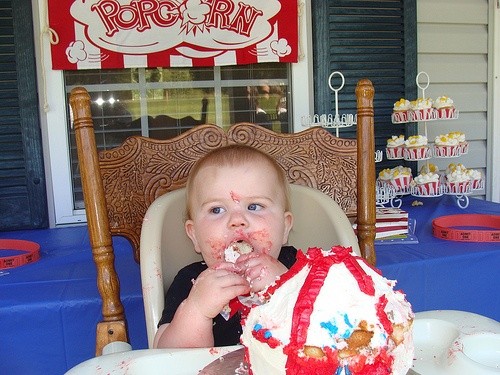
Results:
[238,246,416,375]
[224,242,253,263]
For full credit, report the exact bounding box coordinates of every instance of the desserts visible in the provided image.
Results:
[379,163,481,195]
[385,130,467,160]
[393,96,455,121]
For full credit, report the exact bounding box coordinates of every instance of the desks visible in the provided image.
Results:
[0,195,500,375]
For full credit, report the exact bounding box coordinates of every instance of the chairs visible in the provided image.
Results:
[65,186,499,375]
[69,79,375,352]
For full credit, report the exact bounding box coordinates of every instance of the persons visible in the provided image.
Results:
[153,143,296,348]
